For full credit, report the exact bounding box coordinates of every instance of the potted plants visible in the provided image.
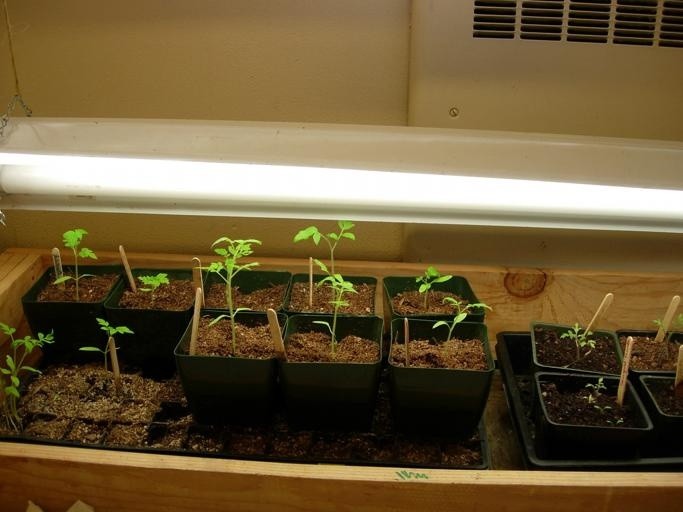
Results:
[21,229,125,368]
[104,268,208,371]
[531,313,683,460]
